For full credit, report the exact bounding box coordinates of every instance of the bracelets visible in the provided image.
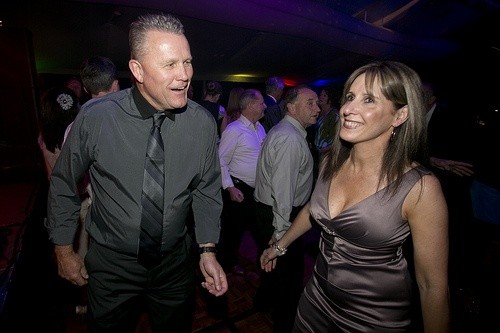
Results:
[199,246,220,255]
[270,242,288,256]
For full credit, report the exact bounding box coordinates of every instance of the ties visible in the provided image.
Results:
[135,110,166,273]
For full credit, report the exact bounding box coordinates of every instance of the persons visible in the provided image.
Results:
[1,14,500,333]
[253,85,321,327]
[260,59,450,333]
[218,88,268,287]
[44,14,229,333]
